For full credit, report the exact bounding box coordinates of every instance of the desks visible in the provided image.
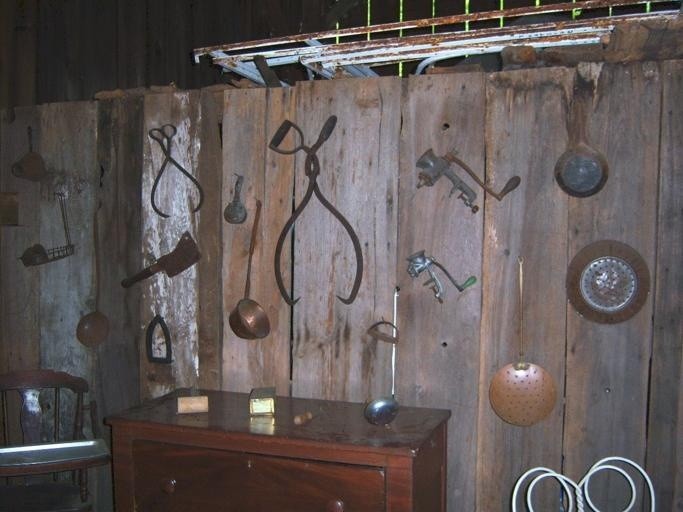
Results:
[101,387,453,511]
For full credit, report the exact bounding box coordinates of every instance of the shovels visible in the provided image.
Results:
[121,239,203,288]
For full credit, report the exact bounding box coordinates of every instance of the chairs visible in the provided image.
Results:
[511,457,656,511]
[0,369,112,512]
